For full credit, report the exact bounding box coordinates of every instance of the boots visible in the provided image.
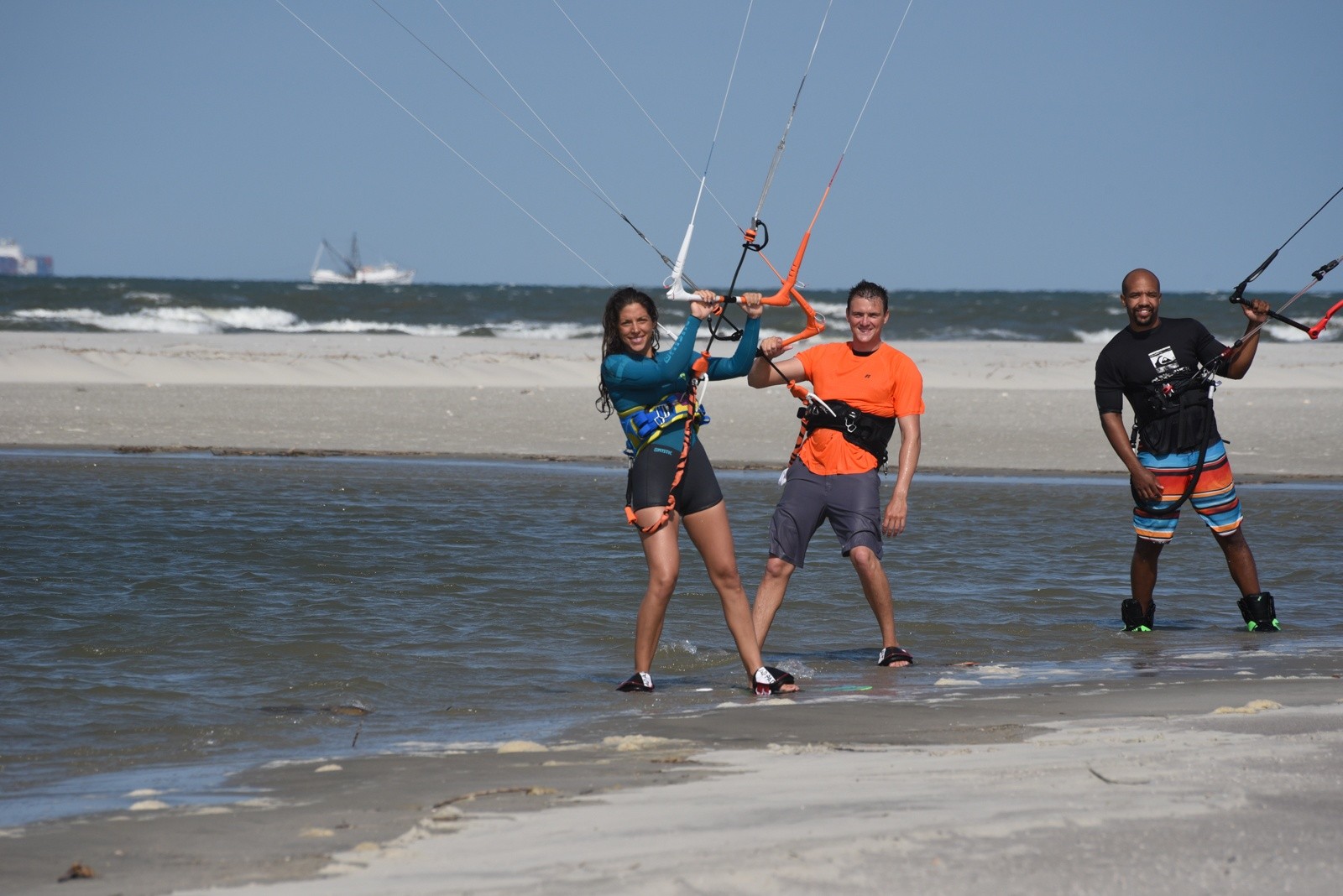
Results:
[1121,599,1156,632]
[1237,592,1281,632]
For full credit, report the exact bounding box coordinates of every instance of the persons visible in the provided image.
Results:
[594,288,803,695]
[1096,269,1281,636]
[748,280,925,669]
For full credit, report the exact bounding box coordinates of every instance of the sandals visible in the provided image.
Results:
[877,647,913,667]
[753,666,795,696]
[616,671,654,692]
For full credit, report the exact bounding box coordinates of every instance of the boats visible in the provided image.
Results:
[311,265,418,287]
